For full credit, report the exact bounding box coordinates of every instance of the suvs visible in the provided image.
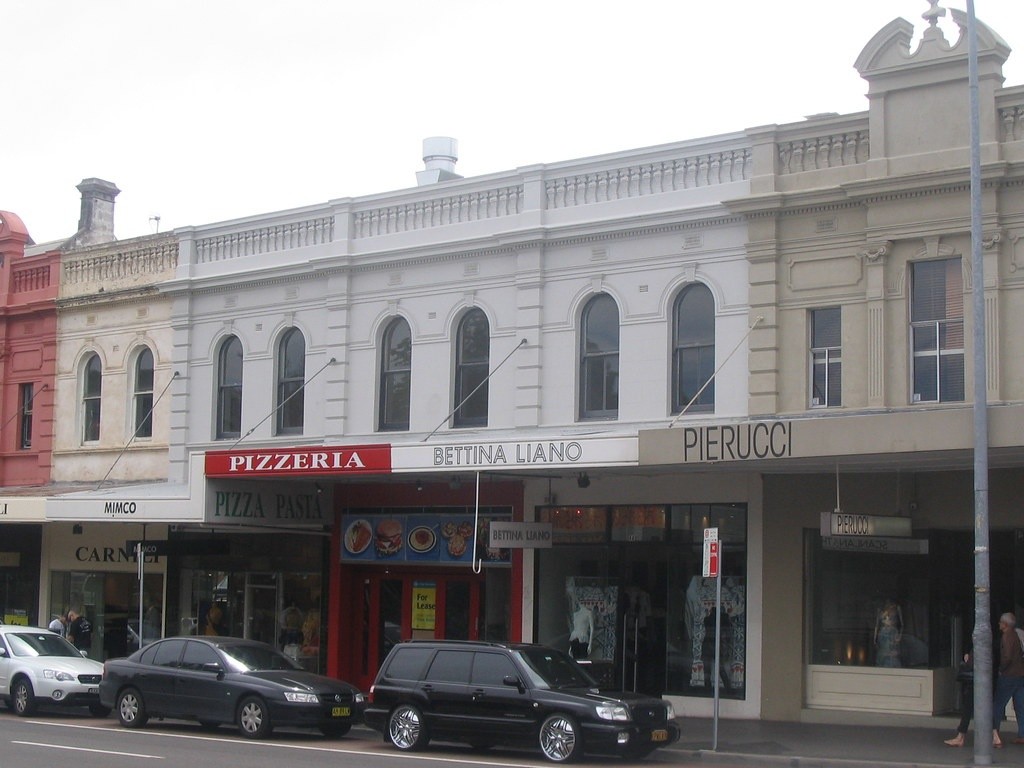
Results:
[126,619,160,653]
[0,625,112,718]
[362,639,681,763]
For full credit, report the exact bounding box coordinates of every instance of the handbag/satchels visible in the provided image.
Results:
[956,654,974,682]
[214,622,227,633]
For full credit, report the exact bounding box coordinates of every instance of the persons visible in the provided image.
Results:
[66,609,94,657]
[702,609,730,692]
[944,646,1001,748]
[205,603,223,636]
[49,613,68,639]
[568,604,593,659]
[994,613,1024,743]
[277,603,304,643]
[874,600,904,667]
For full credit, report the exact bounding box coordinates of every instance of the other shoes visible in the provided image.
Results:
[993,740,1004,748]
[1010,737,1023,743]
[945,739,966,747]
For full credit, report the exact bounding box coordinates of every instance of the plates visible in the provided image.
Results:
[344,519,372,554]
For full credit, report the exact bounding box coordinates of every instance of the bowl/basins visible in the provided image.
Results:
[407,526,436,553]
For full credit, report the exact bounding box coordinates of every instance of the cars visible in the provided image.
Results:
[81,574,96,604]
[98,636,365,739]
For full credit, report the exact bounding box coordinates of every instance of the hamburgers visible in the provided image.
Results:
[374,519,403,554]
